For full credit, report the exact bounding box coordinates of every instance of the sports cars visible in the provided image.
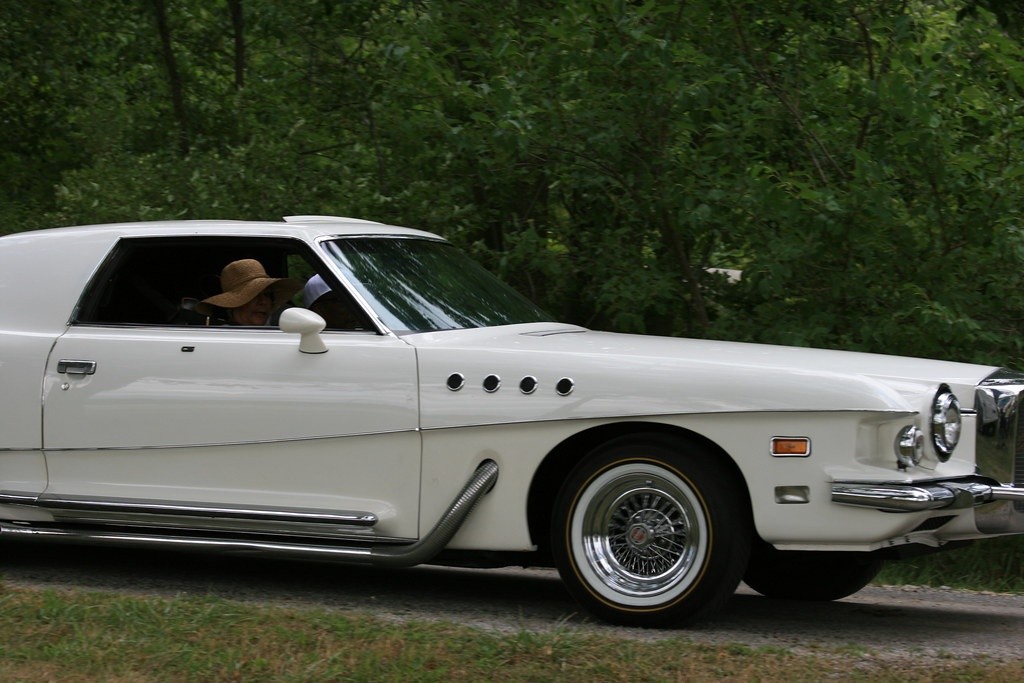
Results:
[0,204,1024,625]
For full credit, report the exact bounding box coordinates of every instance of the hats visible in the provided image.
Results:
[303,273,376,309]
[196,259,302,314]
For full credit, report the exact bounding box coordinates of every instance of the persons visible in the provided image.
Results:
[304,272,352,328]
[196,258,283,329]
